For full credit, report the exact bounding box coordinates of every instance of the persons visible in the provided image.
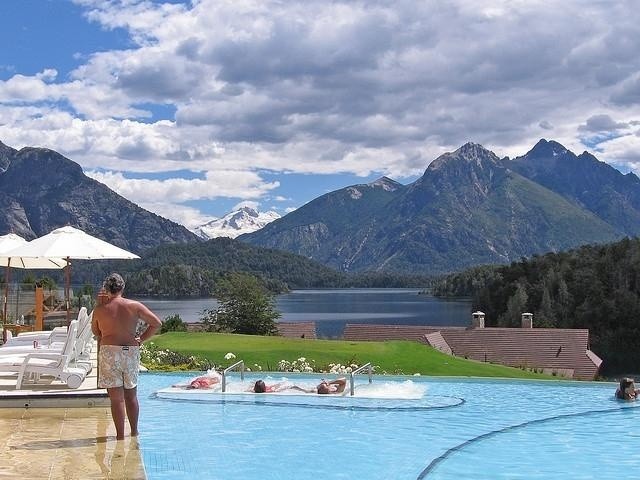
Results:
[634,388,640,396]
[90,273,163,440]
[247,378,285,393]
[172,376,220,391]
[289,377,347,394]
[615,377,635,401]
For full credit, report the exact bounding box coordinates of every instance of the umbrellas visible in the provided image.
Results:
[0,232,71,343]
[2,226,143,334]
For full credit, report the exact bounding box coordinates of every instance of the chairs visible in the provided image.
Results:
[0,306,96,390]
